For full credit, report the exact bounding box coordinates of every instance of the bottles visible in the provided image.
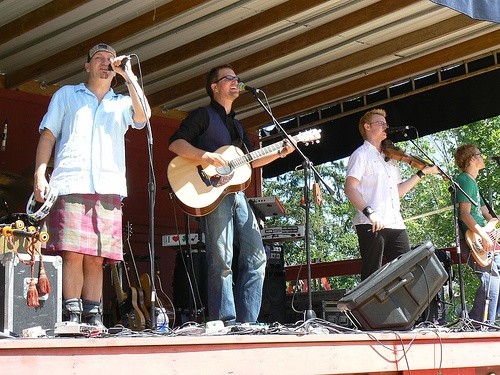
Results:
[156,308,169,332]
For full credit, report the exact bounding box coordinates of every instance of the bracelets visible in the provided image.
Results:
[362,205,374,217]
[125,76,137,85]
[416,170,425,179]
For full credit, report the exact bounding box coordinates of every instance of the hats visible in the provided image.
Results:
[87,43,116,62]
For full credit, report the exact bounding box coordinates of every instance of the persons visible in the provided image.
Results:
[34,44,152,330]
[453,143,500,329]
[344,109,440,282]
[169,64,297,326]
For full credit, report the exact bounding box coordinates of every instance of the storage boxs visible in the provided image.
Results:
[0,252,62,338]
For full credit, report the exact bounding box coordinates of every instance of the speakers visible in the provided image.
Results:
[338,240,448,329]
[173,247,286,323]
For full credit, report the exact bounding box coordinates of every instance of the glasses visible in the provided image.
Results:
[368,120,389,129]
[214,75,240,83]
[472,153,482,157]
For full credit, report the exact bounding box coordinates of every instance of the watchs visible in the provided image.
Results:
[278,148,286,158]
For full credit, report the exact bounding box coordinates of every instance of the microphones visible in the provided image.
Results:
[107,55,130,71]
[488,156,500,160]
[385,127,410,135]
[237,82,260,93]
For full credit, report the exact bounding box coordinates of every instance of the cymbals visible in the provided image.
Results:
[26,186,60,222]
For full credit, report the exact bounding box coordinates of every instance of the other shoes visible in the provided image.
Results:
[82,299,109,335]
[62,298,83,322]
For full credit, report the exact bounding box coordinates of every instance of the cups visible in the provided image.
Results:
[311,258,321,263]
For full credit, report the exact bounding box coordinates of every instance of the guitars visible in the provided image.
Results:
[465,218,500,268]
[167,127,322,217]
[111,263,145,332]
[124,220,175,331]
[121,244,150,329]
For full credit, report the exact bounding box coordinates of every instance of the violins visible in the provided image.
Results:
[383,140,448,182]
